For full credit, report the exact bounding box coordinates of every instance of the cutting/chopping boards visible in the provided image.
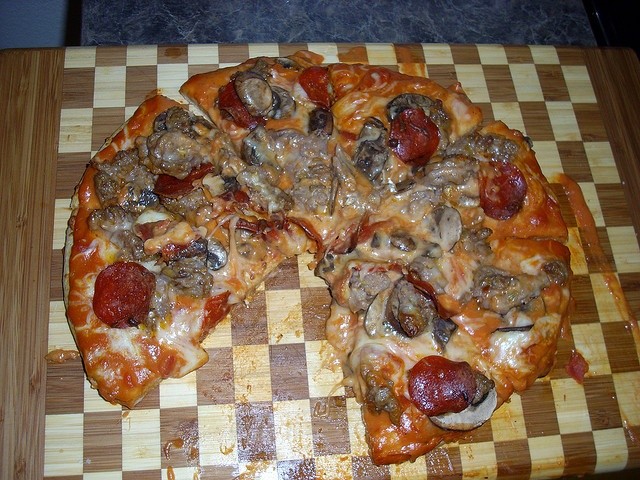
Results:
[2,42,640,479]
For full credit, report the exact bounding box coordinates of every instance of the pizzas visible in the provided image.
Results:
[61,50,572,468]
